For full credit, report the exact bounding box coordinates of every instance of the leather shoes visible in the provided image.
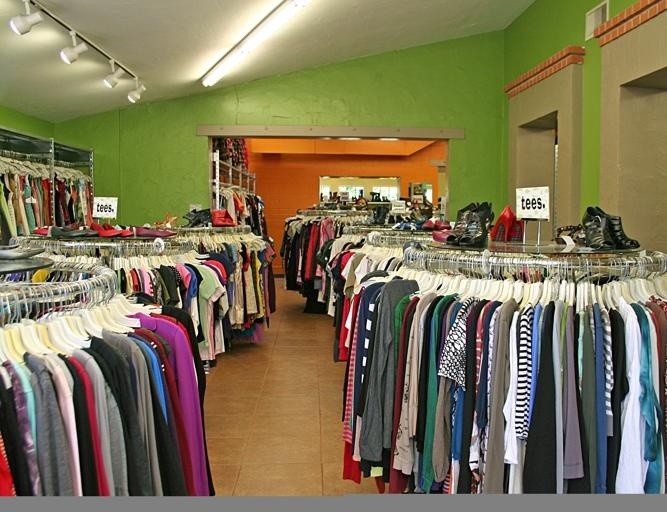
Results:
[432,228,456,244]
[595,205,639,250]
[0,244,45,261]
[434,219,451,230]
[581,206,616,251]
[310,201,367,211]
[421,219,437,231]
[34,221,178,239]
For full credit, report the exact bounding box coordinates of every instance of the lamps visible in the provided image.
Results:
[8,1,146,107]
[190,2,303,90]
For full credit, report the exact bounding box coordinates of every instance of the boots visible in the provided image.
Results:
[181,210,198,228]
[196,209,210,228]
[210,206,236,228]
[375,206,388,224]
[319,189,390,204]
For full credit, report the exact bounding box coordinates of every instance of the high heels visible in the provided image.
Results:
[459,201,495,251]
[446,202,478,244]
[506,215,523,244]
[491,206,513,242]
